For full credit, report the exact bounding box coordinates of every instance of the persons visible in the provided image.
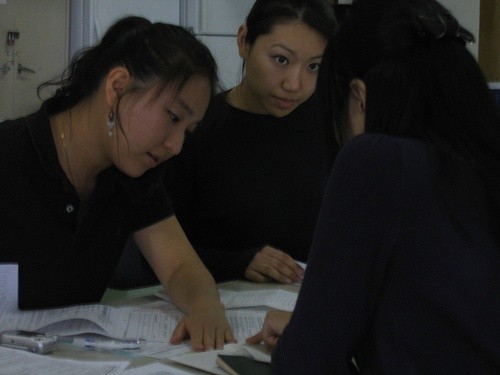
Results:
[0,16,237,355]
[245,0,500,375]
[130,1,350,287]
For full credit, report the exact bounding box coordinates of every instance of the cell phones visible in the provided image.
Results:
[0,330,73,355]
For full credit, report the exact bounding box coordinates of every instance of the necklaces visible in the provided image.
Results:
[55,114,97,206]
[237,80,249,111]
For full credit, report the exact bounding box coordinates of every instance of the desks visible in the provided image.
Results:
[2,280,303,375]
